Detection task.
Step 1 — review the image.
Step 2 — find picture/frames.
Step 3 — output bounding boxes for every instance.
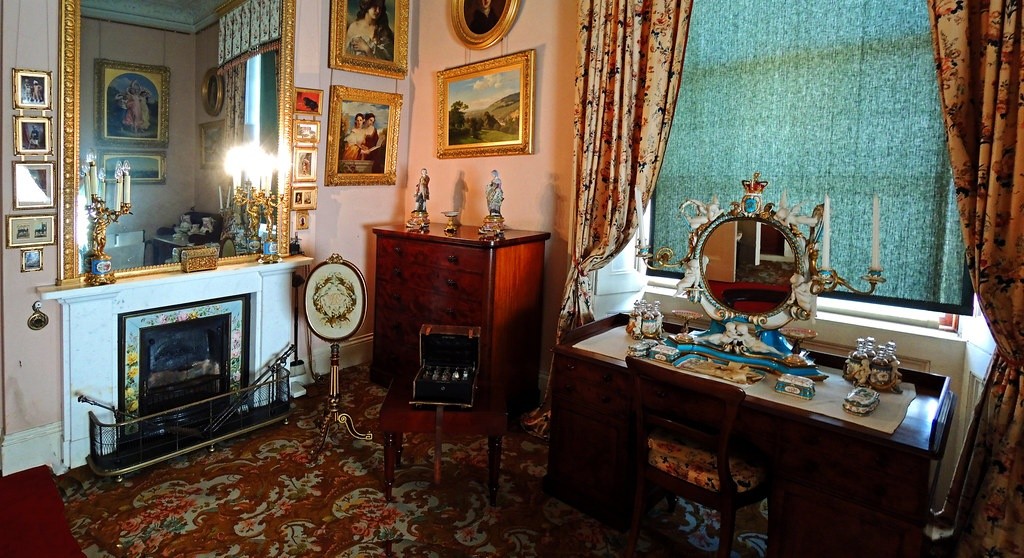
[97,150,167,185]
[201,67,223,116]
[329,0,409,80]
[290,87,324,231]
[434,49,536,160]
[94,58,171,149]
[324,85,404,187]
[449,0,522,50]
[4,68,57,272]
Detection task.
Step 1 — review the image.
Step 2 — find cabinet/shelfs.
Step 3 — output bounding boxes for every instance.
[371,221,552,414]
[153,211,223,265]
[545,310,956,558]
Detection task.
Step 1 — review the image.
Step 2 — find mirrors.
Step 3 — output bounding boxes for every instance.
[673,174,829,330]
[53,0,296,289]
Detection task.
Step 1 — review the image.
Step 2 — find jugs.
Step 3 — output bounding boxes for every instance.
[189,224,200,234]
[180,215,192,232]
[200,217,215,234]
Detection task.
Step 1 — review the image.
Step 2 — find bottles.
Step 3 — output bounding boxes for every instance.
[625,299,664,340]
[842,337,901,389]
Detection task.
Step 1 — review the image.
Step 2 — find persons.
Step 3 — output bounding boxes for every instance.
[302,163,307,174]
[685,204,724,228]
[673,260,700,297]
[414,168,429,212]
[297,193,311,204]
[788,274,816,324]
[633,315,642,334]
[777,202,822,227]
[27,126,39,149]
[23,79,43,102]
[182,192,260,241]
[245,194,276,239]
[890,360,902,382]
[486,171,503,216]
[694,323,784,357]
[856,359,870,384]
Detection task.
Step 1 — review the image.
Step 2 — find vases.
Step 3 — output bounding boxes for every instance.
[199,119,227,170]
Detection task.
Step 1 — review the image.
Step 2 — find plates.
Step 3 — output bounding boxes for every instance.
[441,211,459,217]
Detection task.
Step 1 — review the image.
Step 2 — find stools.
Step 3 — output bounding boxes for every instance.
[380,371,508,507]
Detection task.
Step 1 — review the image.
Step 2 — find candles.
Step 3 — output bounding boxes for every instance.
[219,185,223,210]
[635,186,647,246]
[227,186,230,208]
[871,193,879,268]
[822,194,831,271]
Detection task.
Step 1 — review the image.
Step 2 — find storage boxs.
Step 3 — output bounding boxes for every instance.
[408,323,480,410]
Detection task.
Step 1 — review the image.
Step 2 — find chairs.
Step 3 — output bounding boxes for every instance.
[624,356,768,558]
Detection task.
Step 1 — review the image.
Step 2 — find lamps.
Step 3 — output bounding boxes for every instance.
[79,149,131,286]
[229,155,271,255]
[228,150,284,264]
[82,163,123,274]
